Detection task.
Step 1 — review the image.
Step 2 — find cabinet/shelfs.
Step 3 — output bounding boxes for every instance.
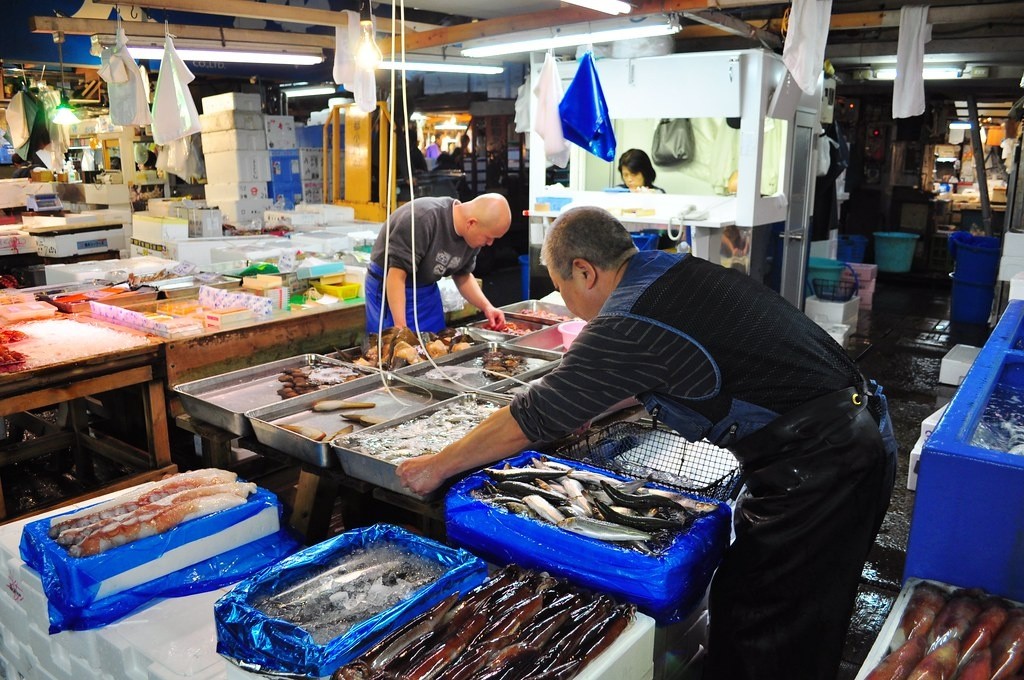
[102,135,170,198]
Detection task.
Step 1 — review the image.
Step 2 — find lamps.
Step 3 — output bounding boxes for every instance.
[281,83,337,97]
[563,0,640,16]
[460,12,683,57]
[52,31,80,125]
[90,33,325,65]
[378,53,504,75]
[870,63,966,78]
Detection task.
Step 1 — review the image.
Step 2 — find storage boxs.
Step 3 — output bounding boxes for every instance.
[0,450,732,680]
[997,229,1024,301]
[855,577,1023,680]
[804,234,878,350]
[908,403,951,491]
[0,92,384,286]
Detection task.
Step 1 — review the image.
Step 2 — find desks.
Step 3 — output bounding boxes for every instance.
[176,410,447,541]
[0,352,178,527]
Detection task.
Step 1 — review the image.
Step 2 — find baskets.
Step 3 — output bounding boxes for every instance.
[309,281,360,300]
[812,279,857,302]
[557,420,745,502]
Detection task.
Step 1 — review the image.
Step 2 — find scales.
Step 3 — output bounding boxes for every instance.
[26,192,64,212]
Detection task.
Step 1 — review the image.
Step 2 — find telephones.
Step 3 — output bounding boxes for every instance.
[666,203,711,222]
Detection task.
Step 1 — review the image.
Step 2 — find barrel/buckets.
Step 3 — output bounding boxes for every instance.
[518,254,530,301]
[804,255,846,297]
[631,232,658,251]
[948,231,1002,281]
[837,234,869,264]
[949,270,998,325]
[874,231,919,273]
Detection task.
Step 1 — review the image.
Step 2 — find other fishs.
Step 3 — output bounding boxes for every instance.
[253,555,436,631]
[955,648,992,680]
[902,583,950,641]
[924,589,983,656]
[863,634,925,680]
[958,605,1010,670]
[330,562,638,680]
[907,637,961,680]
[471,456,718,558]
[989,616,1024,680]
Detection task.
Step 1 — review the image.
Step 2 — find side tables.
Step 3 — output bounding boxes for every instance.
[939,344,982,385]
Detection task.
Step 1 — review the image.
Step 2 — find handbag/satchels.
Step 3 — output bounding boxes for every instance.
[651,118,695,166]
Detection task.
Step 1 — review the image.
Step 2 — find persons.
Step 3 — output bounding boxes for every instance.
[616,149,666,194]
[396,129,477,203]
[365,193,511,347]
[12,153,40,178]
[129,149,165,211]
[395,205,897,680]
